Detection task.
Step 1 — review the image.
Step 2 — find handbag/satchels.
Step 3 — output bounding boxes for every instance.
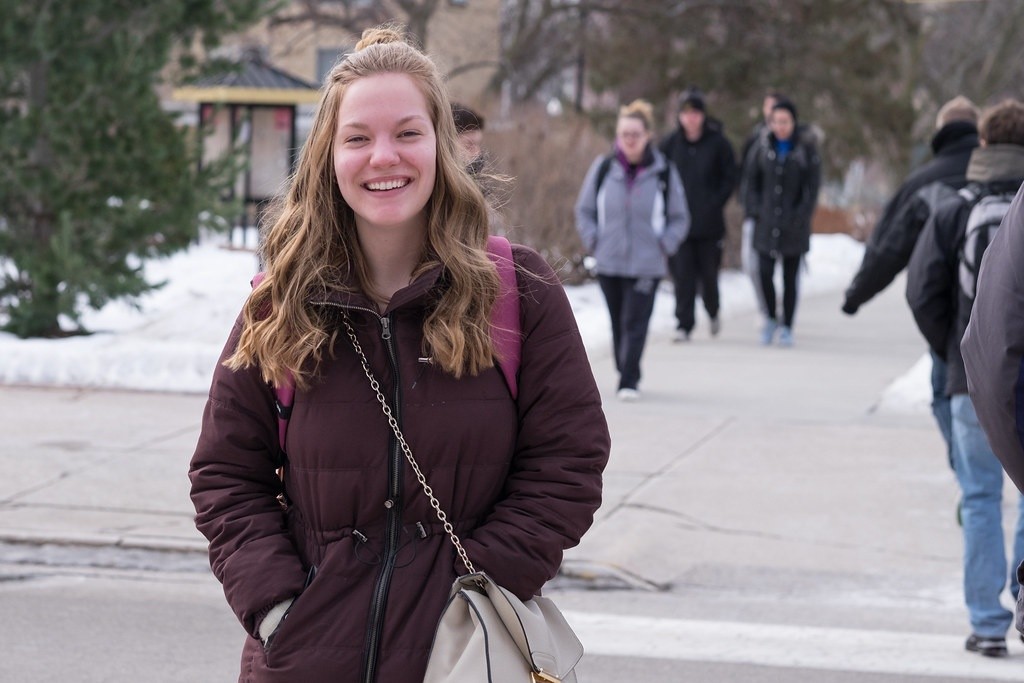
[422,570,584,683]
[736,139,764,203]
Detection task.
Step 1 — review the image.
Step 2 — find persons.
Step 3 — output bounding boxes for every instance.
[188,28,610,682]
[841,91,1024,658]
[447,100,509,243]
[572,87,823,401]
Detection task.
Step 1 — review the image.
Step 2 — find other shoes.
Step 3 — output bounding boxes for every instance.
[619,388,637,400]
[710,315,719,335]
[966,633,1008,657]
[778,323,792,344]
[758,317,777,344]
[957,499,963,526]
[674,328,687,340]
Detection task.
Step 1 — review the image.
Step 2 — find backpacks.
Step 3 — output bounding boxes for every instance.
[958,186,1018,326]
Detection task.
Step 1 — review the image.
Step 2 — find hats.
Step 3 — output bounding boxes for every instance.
[678,89,706,112]
